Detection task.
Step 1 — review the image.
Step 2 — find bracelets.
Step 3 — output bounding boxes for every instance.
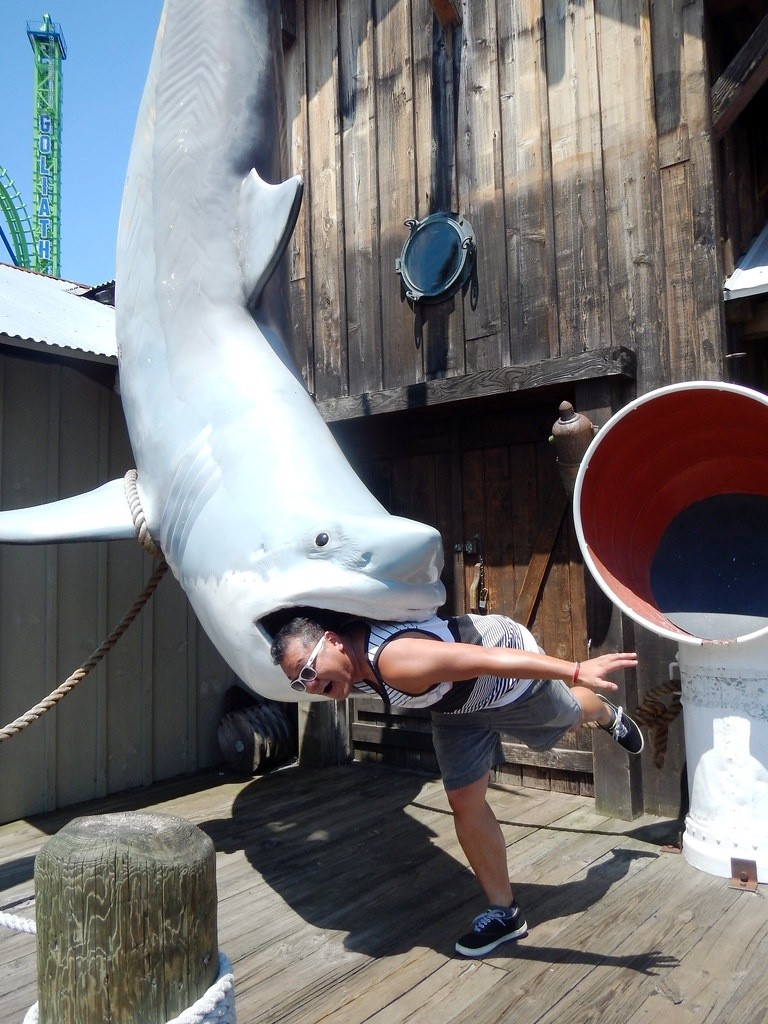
[572,662,580,684]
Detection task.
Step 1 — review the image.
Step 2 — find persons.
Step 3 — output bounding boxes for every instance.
[269,614,645,957]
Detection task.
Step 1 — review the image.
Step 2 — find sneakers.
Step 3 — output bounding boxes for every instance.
[595,692,645,754]
[455,904,529,958]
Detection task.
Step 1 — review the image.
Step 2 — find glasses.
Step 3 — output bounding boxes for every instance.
[290,634,326,695]
